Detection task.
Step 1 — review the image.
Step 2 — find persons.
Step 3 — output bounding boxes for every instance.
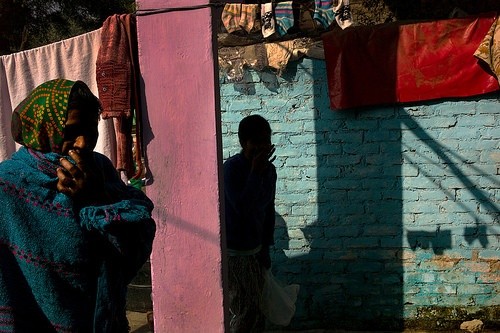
[0,78,157,333]
[223,113,278,333]
